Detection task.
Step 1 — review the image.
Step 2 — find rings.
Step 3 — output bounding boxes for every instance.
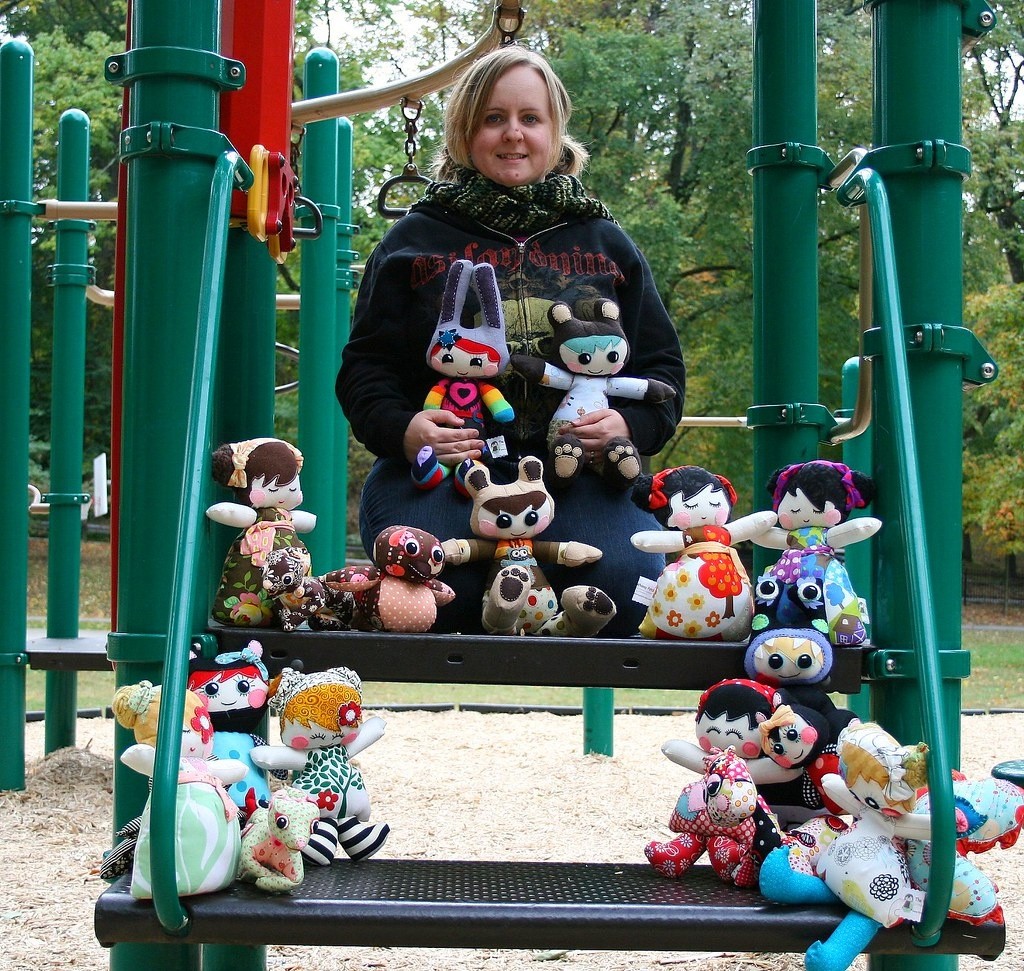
[589,449,597,467]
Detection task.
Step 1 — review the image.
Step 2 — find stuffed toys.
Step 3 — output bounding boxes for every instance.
[205,437,884,642]
[408,259,514,488]
[511,281,678,482]
[645,629,1024,970]
[111,637,392,900]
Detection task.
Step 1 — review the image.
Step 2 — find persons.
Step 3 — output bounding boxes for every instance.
[335,43,686,637]
